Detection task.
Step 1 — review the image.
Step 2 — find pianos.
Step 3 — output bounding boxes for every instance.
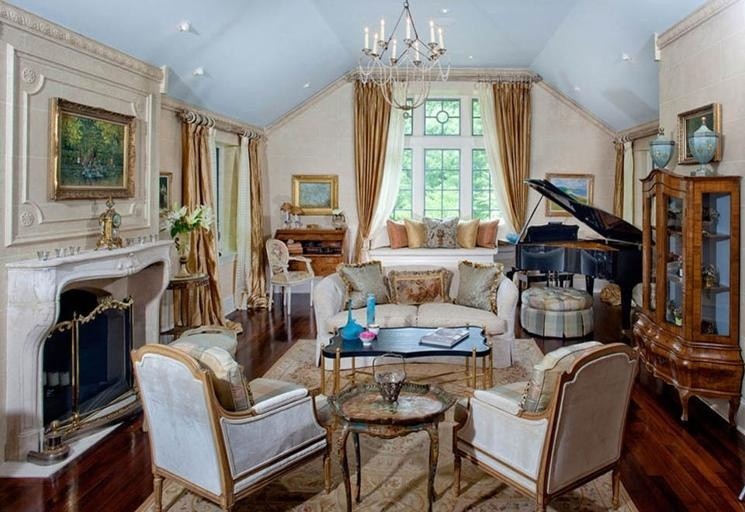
[514,179,655,329]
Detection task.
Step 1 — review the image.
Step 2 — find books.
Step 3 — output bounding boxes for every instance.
[420,327,470,349]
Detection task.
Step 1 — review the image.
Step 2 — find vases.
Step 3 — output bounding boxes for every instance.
[174,258,192,278]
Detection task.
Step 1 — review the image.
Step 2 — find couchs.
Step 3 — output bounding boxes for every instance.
[362,222,513,266]
[314,259,520,370]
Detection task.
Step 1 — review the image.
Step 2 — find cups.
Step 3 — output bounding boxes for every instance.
[38,233,160,262]
[368,324,380,336]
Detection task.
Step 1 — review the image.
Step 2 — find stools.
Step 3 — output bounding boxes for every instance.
[521,287,595,339]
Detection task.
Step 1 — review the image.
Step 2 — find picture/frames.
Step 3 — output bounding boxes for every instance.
[545,173,594,217]
[159,171,173,218]
[292,175,337,215]
[677,103,721,165]
[48,97,137,199]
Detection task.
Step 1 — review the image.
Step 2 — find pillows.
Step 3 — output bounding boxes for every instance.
[336,259,505,315]
[386,216,499,248]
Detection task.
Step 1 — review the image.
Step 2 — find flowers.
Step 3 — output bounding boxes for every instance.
[159,203,215,257]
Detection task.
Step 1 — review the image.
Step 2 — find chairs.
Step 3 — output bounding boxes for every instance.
[453,342,639,511]
[265,239,314,316]
[131,344,334,512]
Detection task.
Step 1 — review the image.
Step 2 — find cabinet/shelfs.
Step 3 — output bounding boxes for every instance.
[633,168,744,428]
[274,229,345,278]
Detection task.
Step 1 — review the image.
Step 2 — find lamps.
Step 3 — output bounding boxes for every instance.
[358,1,451,110]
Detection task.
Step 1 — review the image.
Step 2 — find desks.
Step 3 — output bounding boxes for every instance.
[159,273,210,340]
[326,382,456,512]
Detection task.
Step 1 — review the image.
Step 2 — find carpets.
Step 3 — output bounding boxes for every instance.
[136,339,638,512]
